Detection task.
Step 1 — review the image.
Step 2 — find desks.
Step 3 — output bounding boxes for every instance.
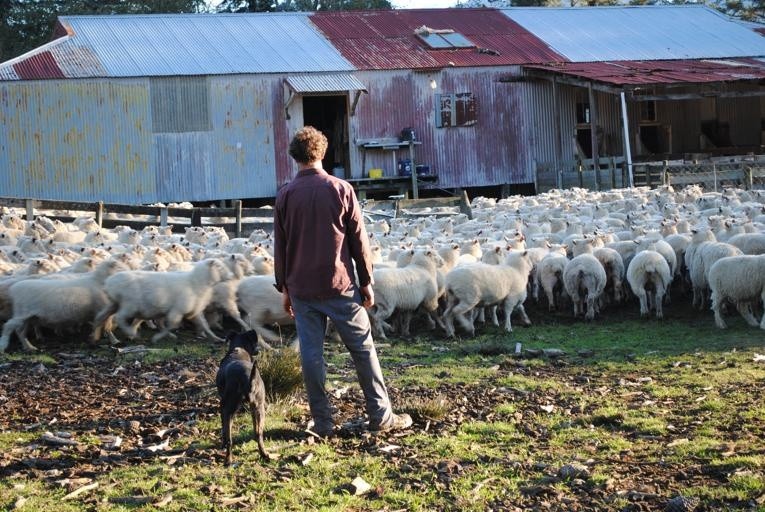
[344,174,439,202]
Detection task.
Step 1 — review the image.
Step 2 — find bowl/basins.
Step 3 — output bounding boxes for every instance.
[369,169,382,178]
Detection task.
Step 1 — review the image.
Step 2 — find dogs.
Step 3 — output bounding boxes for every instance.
[214,328,278,467]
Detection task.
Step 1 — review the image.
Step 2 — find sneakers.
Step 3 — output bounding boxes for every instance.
[370,413,412,434]
[310,426,333,439]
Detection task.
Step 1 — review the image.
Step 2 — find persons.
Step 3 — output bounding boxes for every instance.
[272,126,413,437]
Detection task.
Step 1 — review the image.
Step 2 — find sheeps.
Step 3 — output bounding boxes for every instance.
[1,183,765,353]
